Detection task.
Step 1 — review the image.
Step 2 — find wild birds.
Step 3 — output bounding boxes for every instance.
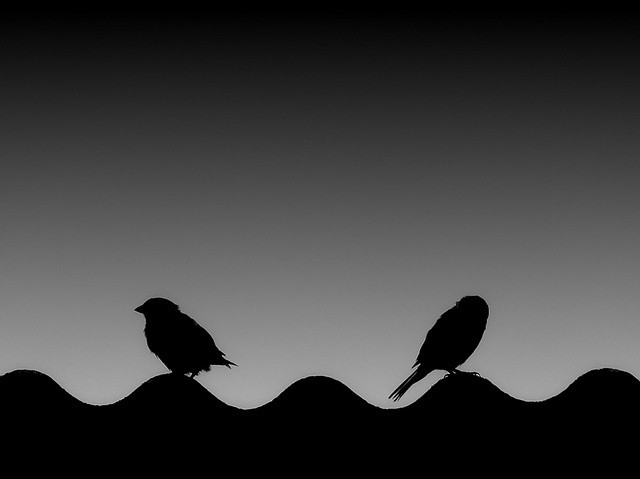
[388,295,489,402]
[133,297,239,379]
